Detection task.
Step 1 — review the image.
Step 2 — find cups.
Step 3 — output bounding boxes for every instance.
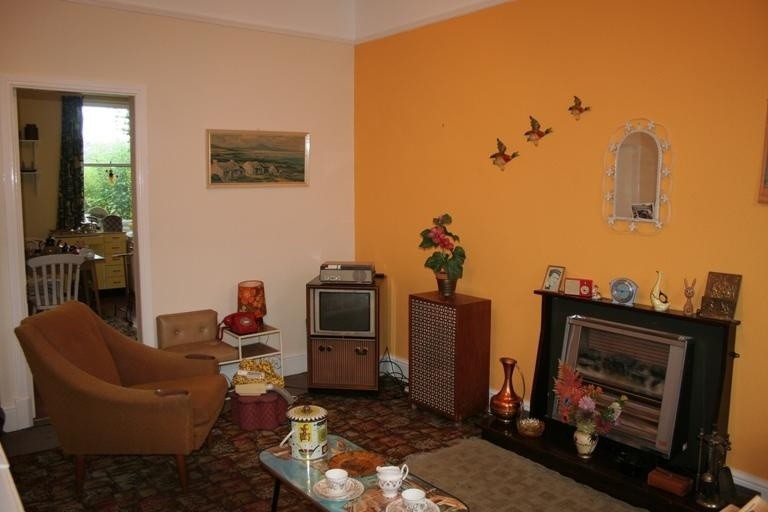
[325,467,347,496]
[402,488,427,512]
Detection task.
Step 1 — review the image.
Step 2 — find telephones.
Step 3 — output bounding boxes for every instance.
[223,311,258,335]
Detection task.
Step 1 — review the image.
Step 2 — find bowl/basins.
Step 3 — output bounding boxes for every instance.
[516,415,545,439]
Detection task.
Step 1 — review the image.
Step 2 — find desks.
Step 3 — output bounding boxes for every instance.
[26,248,103,318]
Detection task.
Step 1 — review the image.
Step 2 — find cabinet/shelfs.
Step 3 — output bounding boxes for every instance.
[305,274,381,393]
[19,138,39,176]
[49,232,129,291]
[406,291,492,425]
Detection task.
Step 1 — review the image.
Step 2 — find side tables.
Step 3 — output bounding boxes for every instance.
[220,323,285,385]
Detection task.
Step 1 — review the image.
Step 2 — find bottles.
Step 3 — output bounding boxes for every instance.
[56,241,80,256]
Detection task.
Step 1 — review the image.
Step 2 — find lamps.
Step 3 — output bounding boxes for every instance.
[238,279,267,326]
[693,421,732,508]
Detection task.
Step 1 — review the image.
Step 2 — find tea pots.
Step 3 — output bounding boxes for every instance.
[376,464,409,499]
[38,236,56,255]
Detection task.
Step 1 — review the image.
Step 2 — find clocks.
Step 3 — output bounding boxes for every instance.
[608,278,639,308]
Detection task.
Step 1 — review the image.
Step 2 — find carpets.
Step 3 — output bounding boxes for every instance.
[398,436,642,511]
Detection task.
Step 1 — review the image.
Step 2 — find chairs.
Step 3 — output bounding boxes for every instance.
[25,237,47,256]
[27,254,86,315]
[156,308,240,390]
[14,300,226,503]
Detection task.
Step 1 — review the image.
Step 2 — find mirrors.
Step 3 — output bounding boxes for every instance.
[601,118,671,233]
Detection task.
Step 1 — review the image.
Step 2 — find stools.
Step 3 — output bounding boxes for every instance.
[232,390,290,434]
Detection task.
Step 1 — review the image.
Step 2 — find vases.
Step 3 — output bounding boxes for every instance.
[487,356,524,422]
[574,420,600,459]
[432,269,459,299]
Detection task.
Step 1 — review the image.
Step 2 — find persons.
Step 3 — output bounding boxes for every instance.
[543,269,560,291]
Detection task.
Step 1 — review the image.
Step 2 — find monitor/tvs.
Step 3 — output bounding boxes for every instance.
[310,287,376,339]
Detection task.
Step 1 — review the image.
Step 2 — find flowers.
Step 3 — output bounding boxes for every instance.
[419,212,467,279]
[553,360,629,437]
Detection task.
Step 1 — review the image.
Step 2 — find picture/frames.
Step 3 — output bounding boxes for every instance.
[207,128,311,188]
[540,264,566,295]
[757,99,768,204]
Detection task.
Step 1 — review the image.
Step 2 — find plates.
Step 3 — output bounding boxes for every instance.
[311,477,364,501]
[383,497,442,512]
